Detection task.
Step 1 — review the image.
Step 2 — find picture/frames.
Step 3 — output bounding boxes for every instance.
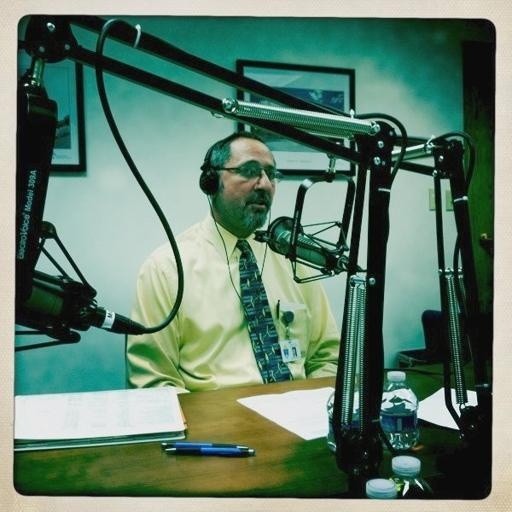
[17,41,90,176]
[234,57,357,179]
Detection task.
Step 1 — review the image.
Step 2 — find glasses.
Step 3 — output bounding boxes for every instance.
[214,167,276,179]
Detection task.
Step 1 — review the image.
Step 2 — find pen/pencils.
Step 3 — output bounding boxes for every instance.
[161,442,255,456]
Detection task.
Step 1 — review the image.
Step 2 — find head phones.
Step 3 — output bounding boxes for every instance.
[199,146,219,195]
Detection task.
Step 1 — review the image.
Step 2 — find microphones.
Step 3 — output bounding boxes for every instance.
[266,216,338,270]
[14,268,96,342]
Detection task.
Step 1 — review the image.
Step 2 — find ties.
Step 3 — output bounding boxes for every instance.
[237,240,290,384]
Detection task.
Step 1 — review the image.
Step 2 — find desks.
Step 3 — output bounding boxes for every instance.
[13,365,492,500]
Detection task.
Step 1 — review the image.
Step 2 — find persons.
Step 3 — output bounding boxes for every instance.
[125,131,343,395]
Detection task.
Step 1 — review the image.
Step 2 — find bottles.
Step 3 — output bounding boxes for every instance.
[327,372,363,453]
[367,454,434,500]
[379,370,422,451]
